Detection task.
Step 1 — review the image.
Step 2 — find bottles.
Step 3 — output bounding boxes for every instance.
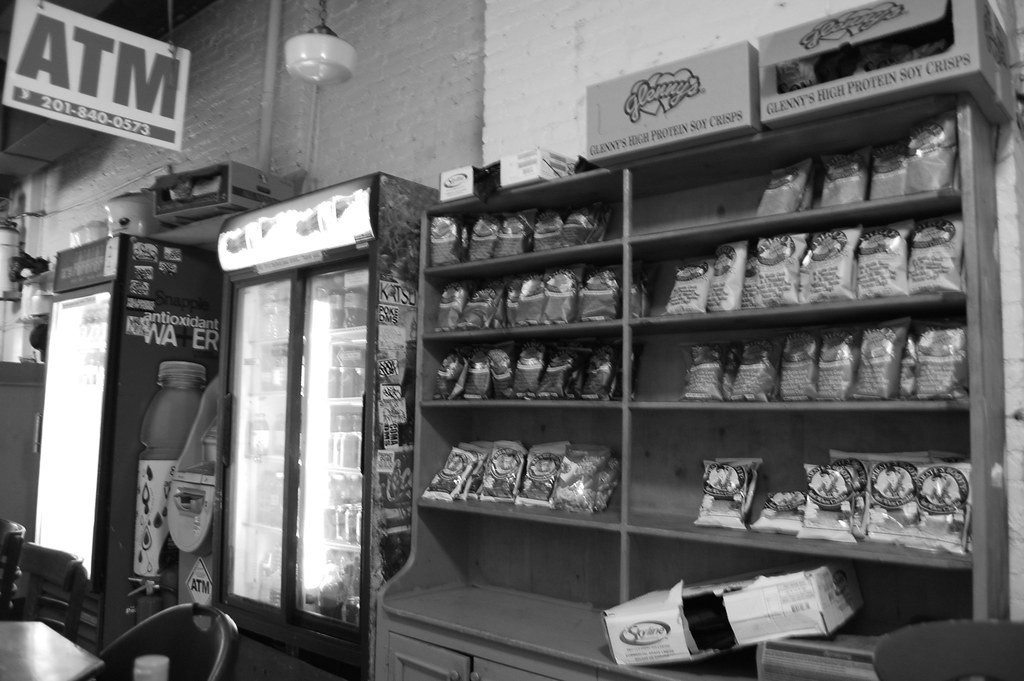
[132,361,206,597]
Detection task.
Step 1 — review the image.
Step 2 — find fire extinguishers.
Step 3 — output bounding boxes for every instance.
[127,576,177,624]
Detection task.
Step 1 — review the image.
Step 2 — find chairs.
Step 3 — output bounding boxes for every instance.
[88,601,240,681]
[9,542,87,643]
[0,516,27,620]
[872,617,1024,681]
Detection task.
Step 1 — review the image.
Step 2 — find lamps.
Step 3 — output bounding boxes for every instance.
[284,0,361,86]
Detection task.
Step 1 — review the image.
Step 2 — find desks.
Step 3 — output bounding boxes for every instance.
[0,620,106,681]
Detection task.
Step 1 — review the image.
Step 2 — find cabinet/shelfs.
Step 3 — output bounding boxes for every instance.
[368,90,1011,681]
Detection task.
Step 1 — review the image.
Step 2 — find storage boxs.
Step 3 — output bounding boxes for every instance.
[601,558,865,666]
[148,159,295,227]
[439,164,498,203]
[753,618,889,681]
[586,39,761,168]
[758,0,1016,127]
[500,146,579,189]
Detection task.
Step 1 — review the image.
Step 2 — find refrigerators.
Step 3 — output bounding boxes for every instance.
[34,233,220,653]
[217,172,438,681]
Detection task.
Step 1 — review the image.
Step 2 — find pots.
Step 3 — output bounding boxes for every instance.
[104,192,155,237]
